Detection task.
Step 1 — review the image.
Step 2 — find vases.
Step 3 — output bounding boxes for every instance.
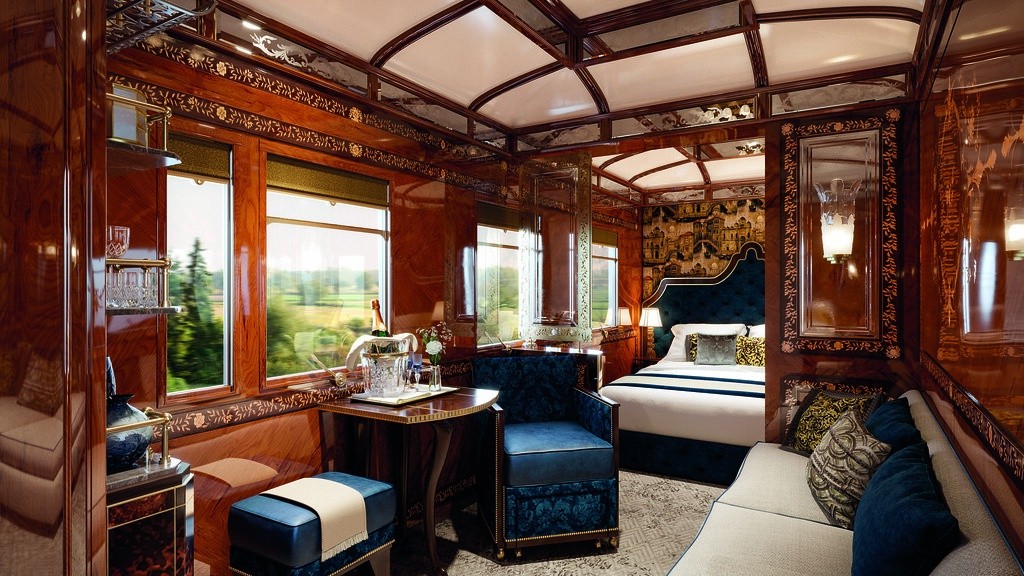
[428,364,440,391]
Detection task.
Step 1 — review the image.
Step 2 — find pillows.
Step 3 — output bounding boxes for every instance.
[849,440,970,576]
[863,396,925,457]
[778,384,876,460]
[665,323,765,368]
[802,406,891,529]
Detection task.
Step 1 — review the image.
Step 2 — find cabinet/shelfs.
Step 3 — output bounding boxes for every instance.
[104,134,198,576]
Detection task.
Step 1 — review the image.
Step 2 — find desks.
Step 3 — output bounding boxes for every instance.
[314,380,500,567]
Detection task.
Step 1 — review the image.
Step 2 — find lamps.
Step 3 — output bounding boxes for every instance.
[810,175,863,262]
[617,306,632,325]
[638,307,663,360]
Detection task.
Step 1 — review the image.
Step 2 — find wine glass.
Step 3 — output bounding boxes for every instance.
[405,351,414,388]
[412,354,422,392]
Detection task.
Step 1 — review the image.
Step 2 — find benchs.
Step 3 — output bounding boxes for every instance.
[226,470,396,576]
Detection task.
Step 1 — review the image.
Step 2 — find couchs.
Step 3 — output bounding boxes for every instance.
[664,388,1022,576]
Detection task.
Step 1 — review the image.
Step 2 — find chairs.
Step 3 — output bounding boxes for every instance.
[469,352,621,563]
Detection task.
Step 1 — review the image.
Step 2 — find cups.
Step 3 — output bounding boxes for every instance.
[106,225,130,257]
[106,271,158,308]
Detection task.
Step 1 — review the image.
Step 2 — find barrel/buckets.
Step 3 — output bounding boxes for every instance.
[360,351,407,396]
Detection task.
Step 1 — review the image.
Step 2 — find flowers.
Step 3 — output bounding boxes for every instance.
[415,320,451,386]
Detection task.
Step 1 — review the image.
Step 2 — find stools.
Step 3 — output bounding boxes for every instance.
[188,456,278,513]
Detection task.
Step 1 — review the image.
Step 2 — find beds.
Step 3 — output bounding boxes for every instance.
[595,242,765,459]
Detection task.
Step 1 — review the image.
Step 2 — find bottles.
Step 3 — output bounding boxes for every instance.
[370,299,394,353]
